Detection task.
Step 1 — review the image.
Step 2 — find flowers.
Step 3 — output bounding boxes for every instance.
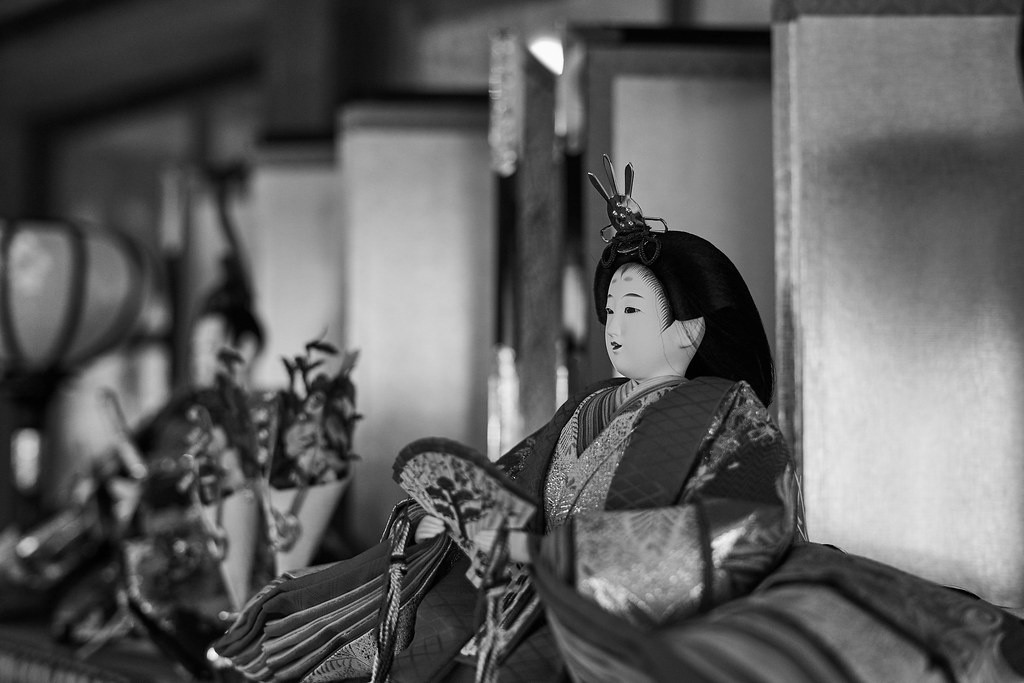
[183,331,363,567]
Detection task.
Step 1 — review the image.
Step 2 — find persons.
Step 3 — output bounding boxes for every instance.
[208,231,1024,683]
